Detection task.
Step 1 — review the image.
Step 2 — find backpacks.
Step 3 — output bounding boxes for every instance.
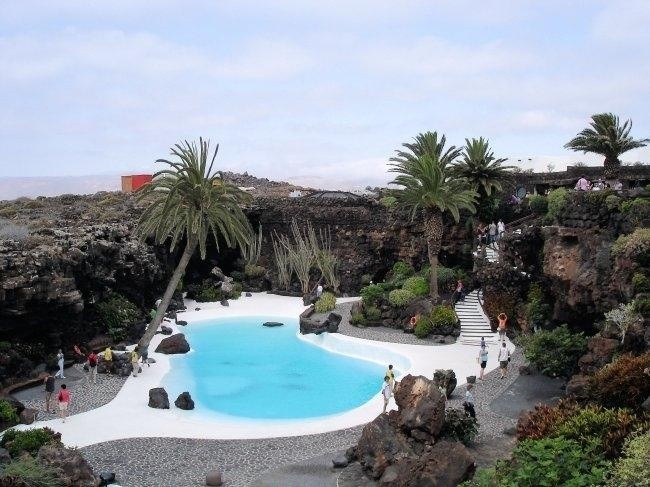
[89,355,96,367]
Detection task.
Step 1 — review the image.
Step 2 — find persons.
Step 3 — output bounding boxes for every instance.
[456,279,464,301]
[142,342,150,367]
[143,297,163,331]
[317,283,323,296]
[72,343,98,384]
[53,349,66,379]
[57,384,70,423]
[46,371,56,413]
[477,311,511,381]
[104,342,113,377]
[505,193,521,205]
[574,173,623,197]
[382,365,397,415]
[465,384,478,423]
[131,346,140,378]
[477,218,506,244]
[431,375,450,420]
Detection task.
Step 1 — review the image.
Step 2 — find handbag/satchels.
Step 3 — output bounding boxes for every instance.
[59,392,62,401]
